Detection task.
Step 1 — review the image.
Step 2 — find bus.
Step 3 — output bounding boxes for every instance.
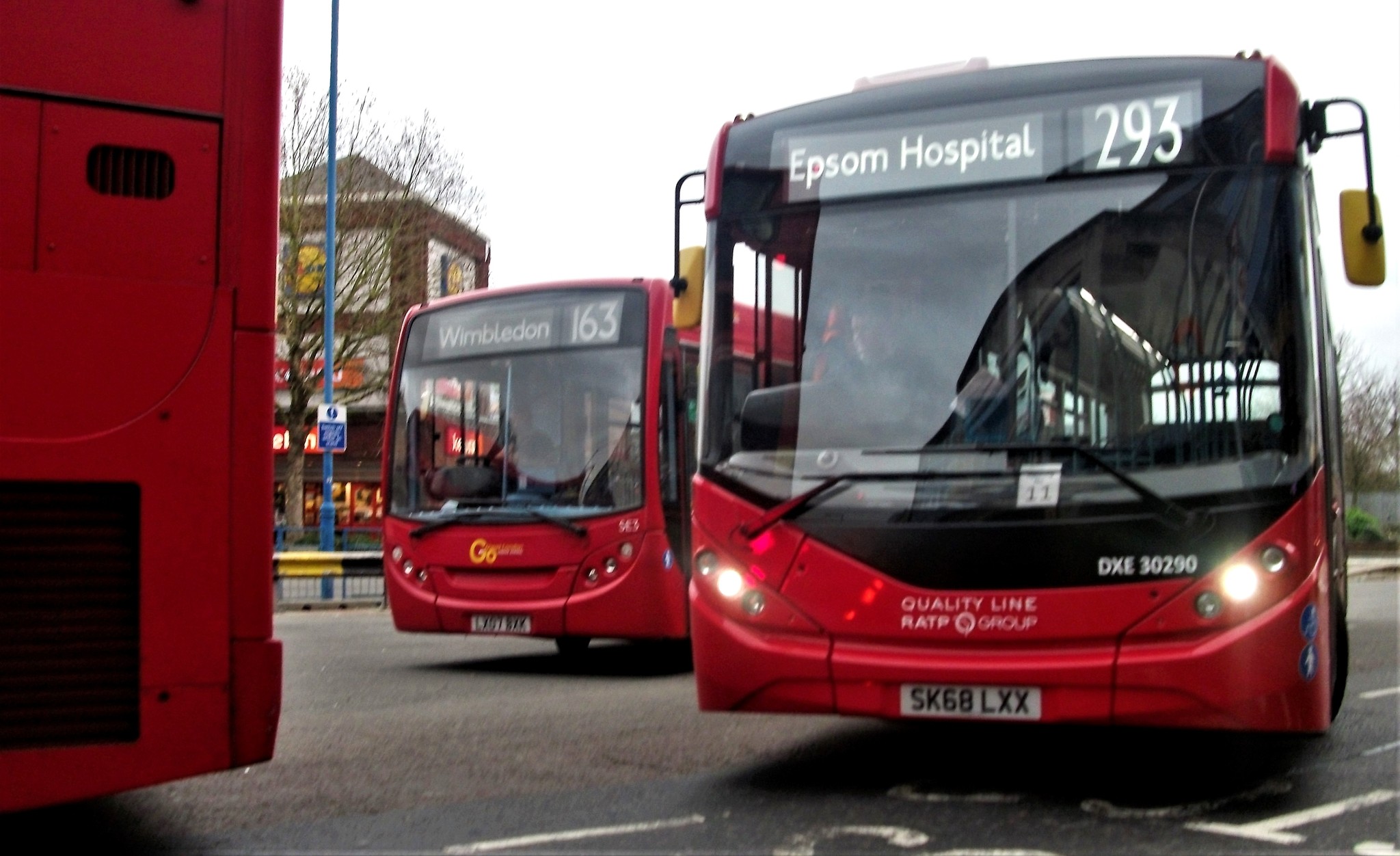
[380,277,809,656]
[0,0,284,813]
[668,48,1388,736]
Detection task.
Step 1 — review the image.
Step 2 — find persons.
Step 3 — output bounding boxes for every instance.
[828,296,954,447]
[482,355,564,497]
